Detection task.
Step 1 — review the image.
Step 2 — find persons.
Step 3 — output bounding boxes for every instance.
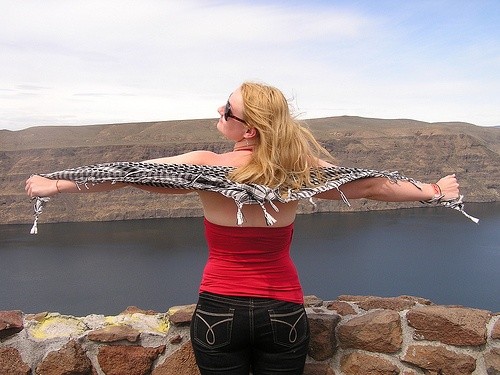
[23,82,461,375]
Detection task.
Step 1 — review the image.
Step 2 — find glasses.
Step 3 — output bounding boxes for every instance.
[224,99,247,126]
